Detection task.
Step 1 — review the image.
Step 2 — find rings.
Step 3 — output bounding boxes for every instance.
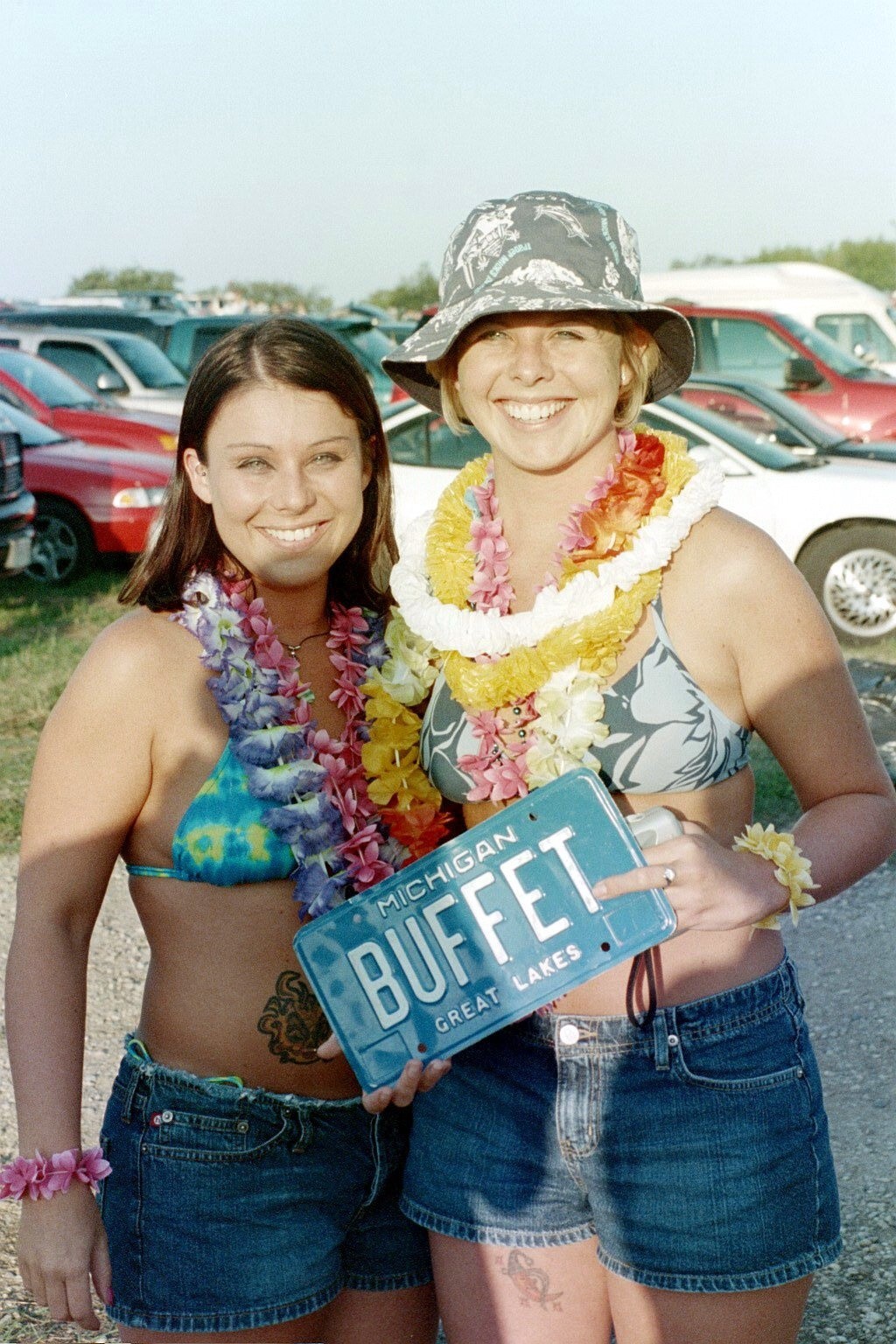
[663,865,677,889]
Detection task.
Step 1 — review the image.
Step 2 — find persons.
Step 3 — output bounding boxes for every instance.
[384,191,896,1344]
[2,318,442,1344]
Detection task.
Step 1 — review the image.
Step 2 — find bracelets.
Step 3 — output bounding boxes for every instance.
[732,821,821,931]
[0,1147,113,1202]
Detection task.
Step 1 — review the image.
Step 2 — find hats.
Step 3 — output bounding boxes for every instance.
[380,191,695,425]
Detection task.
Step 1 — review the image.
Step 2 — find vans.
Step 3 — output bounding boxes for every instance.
[654,256,895,381]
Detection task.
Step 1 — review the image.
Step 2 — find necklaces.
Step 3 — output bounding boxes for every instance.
[280,629,331,659]
[386,418,724,806]
[173,549,454,916]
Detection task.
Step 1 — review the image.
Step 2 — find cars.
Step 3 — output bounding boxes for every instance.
[1,287,428,412]
[1,344,187,590]
[388,369,895,464]
[419,294,895,450]
[374,392,895,651]
